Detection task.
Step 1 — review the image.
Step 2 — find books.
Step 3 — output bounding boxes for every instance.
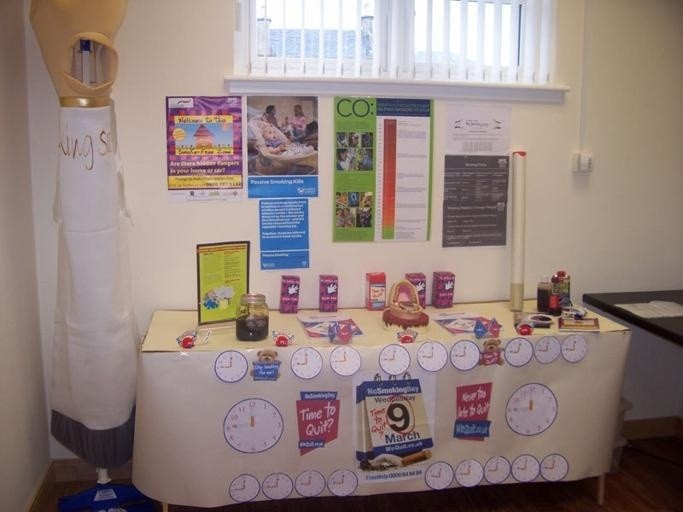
[557,315,600,332]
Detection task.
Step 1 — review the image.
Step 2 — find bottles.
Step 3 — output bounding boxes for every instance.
[236,293,270,342]
[536,269,571,316]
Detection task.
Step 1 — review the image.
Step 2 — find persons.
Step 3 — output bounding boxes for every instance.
[260,126,314,158]
[283,103,308,139]
[260,103,278,128]
[336,208,344,227]
[338,207,355,229]
[336,148,349,171]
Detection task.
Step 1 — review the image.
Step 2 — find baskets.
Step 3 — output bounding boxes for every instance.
[390,280,422,320]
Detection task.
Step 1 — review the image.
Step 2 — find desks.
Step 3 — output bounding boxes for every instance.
[131,299,632,512]
[583,290,683,348]
